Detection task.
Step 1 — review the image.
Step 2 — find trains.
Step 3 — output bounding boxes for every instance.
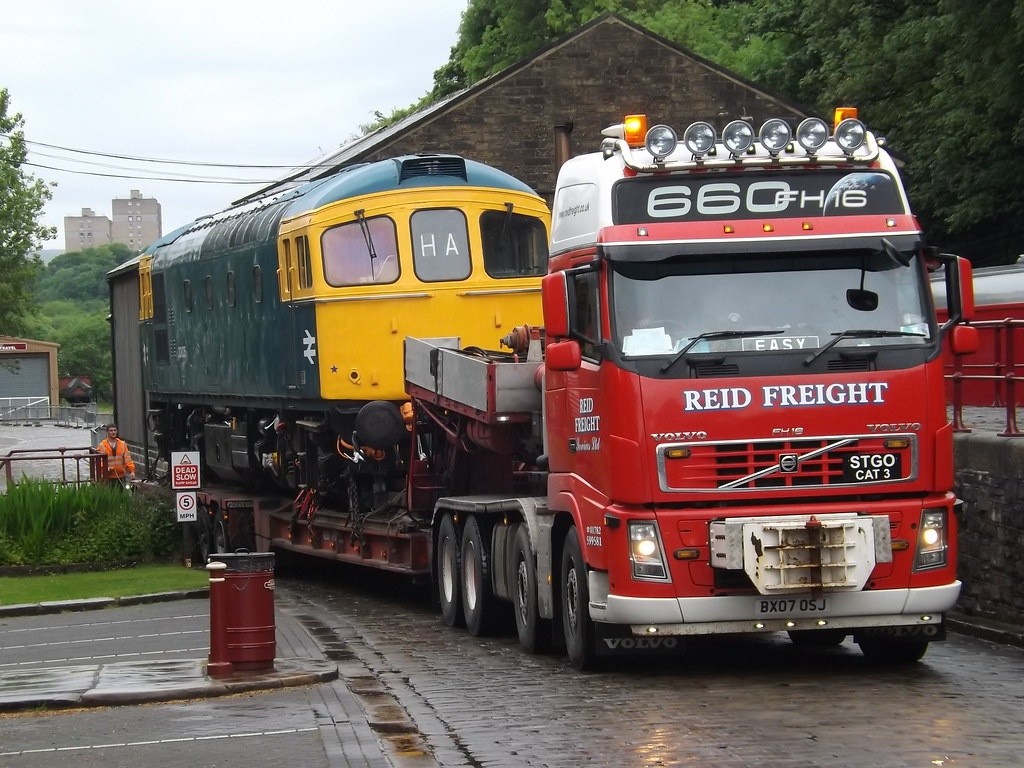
[135,148,552,516]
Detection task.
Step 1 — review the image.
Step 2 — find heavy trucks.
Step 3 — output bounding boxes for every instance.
[139,105,979,678]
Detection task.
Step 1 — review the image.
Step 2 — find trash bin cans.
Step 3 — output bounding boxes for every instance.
[208,547,277,678]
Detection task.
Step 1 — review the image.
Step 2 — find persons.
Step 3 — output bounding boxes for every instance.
[96,423,136,493]
[636,318,649,328]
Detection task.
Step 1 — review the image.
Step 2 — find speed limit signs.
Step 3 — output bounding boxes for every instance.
[177,492,198,522]
